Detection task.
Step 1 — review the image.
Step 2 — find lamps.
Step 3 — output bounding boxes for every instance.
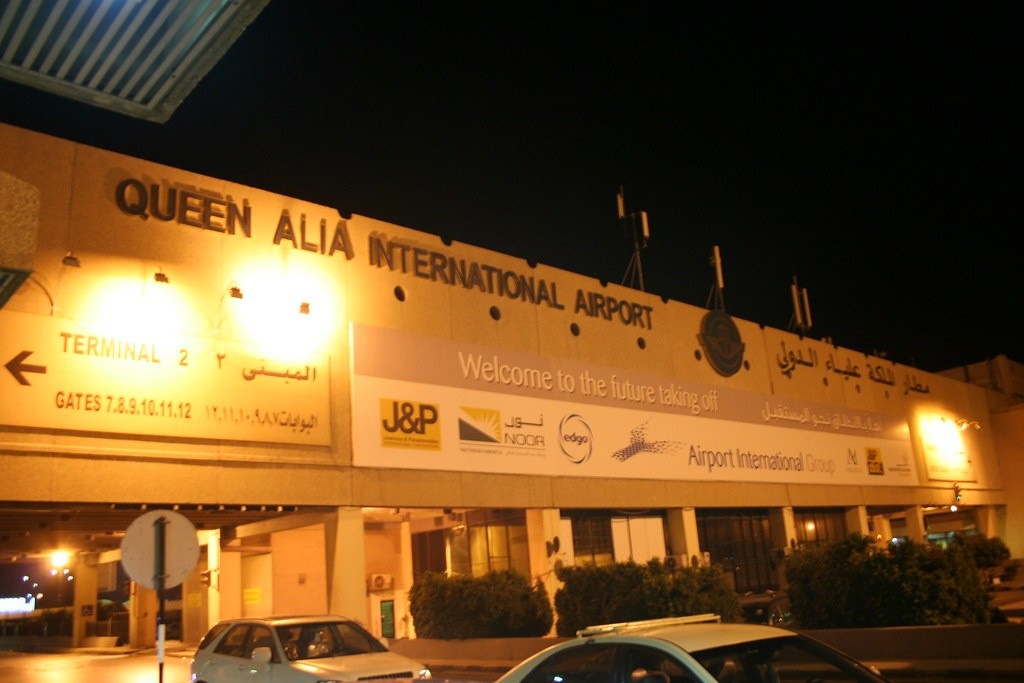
[155,272,169,282]
[62,256,81,267]
[230,288,243,299]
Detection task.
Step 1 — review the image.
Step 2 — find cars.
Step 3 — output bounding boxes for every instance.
[190,614,433,683]
[495,614,897,682]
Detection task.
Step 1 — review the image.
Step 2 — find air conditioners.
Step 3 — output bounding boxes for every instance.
[770,546,789,563]
[666,553,687,568]
[372,574,392,590]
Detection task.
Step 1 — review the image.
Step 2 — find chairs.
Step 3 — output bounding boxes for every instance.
[305,631,330,658]
[274,632,298,663]
